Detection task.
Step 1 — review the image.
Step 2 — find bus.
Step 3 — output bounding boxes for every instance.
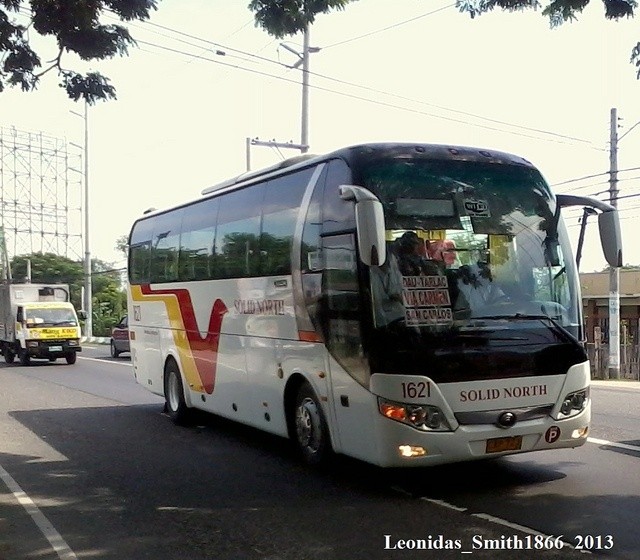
[127,142,622,471]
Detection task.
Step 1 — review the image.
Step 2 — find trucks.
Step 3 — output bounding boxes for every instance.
[1,284,81,367]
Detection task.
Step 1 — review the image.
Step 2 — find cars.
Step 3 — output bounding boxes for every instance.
[109,312,130,358]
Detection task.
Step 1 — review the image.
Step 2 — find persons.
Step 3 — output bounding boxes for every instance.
[395,231,430,276]
[369,253,420,334]
[453,263,510,311]
